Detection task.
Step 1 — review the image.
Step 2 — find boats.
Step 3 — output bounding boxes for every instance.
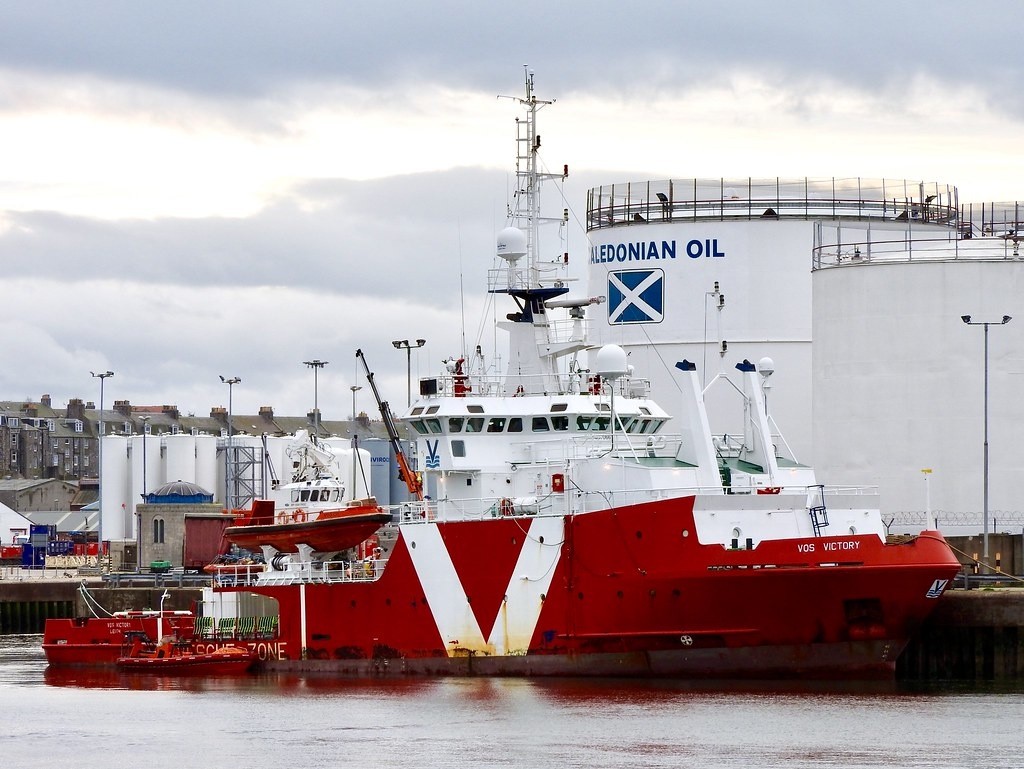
[115,588,261,675]
[222,424,393,553]
[202,63,962,676]
[42,608,196,667]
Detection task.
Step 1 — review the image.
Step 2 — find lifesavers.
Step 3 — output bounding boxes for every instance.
[278,510,288,524]
[501,498,514,516]
[292,509,305,523]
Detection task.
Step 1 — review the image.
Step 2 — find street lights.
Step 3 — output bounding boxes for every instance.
[392,339,426,501]
[963,314,1011,569]
[302,359,329,478]
[218,375,240,515]
[350,386,362,500]
[139,416,152,504]
[90,371,114,554]
